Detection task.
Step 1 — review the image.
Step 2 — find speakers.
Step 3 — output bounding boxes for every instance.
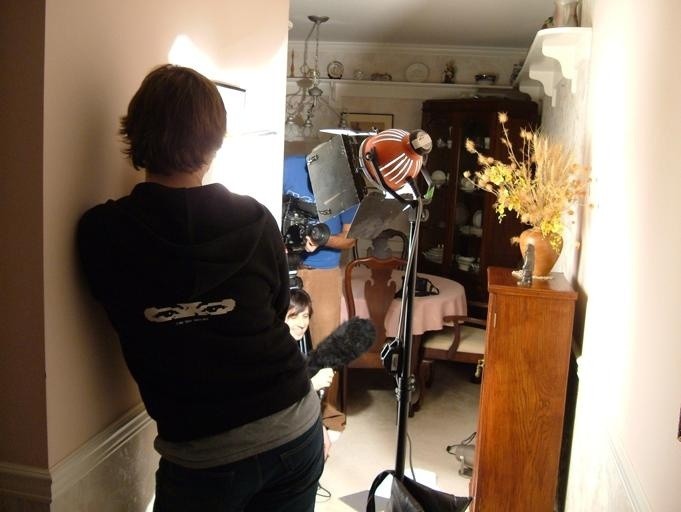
[304,135,363,223]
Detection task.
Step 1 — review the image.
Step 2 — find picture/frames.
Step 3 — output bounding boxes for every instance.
[340,112,397,133]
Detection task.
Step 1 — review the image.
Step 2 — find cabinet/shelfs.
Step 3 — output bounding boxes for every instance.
[466,266,580,511]
[417,98,540,329]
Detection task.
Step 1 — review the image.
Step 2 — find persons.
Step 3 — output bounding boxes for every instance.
[73,62,325,511]
[283,154,361,443]
[284,288,336,464]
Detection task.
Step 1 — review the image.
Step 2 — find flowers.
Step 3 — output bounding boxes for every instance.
[464,106,597,260]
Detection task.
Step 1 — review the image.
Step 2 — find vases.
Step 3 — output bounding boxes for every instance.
[517,228,565,278]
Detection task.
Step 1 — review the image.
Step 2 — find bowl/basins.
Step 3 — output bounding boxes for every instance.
[474,74,497,85]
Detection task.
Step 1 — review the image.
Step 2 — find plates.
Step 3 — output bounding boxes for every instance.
[326,61,344,79]
[405,63,427,83]
[455,203,482,236]
[425,247,444,261]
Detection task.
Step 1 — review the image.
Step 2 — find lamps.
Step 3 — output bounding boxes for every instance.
[360,126,440,511]
[284,14,352,138]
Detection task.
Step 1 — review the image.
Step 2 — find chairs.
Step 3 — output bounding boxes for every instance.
[341,228,486,427]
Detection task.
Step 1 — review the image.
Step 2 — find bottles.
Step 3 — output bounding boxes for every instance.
[521,244,535,287]
